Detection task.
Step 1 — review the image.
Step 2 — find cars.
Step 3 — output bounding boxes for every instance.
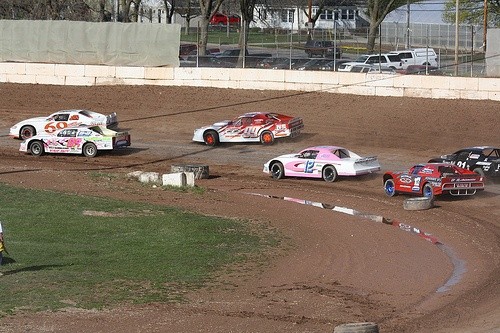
[179,43,404,75]
[262,146,382,183]
[427,146,500,183]
[382,162,486,201]
[192,111,305,147]
[19,123,131,157]
[209,11,241,27]
[8,108,119,140]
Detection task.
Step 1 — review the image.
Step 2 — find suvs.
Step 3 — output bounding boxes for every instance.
[304,39,341,59]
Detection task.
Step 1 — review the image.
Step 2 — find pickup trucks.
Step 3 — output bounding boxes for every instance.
[387,47,438,70]
